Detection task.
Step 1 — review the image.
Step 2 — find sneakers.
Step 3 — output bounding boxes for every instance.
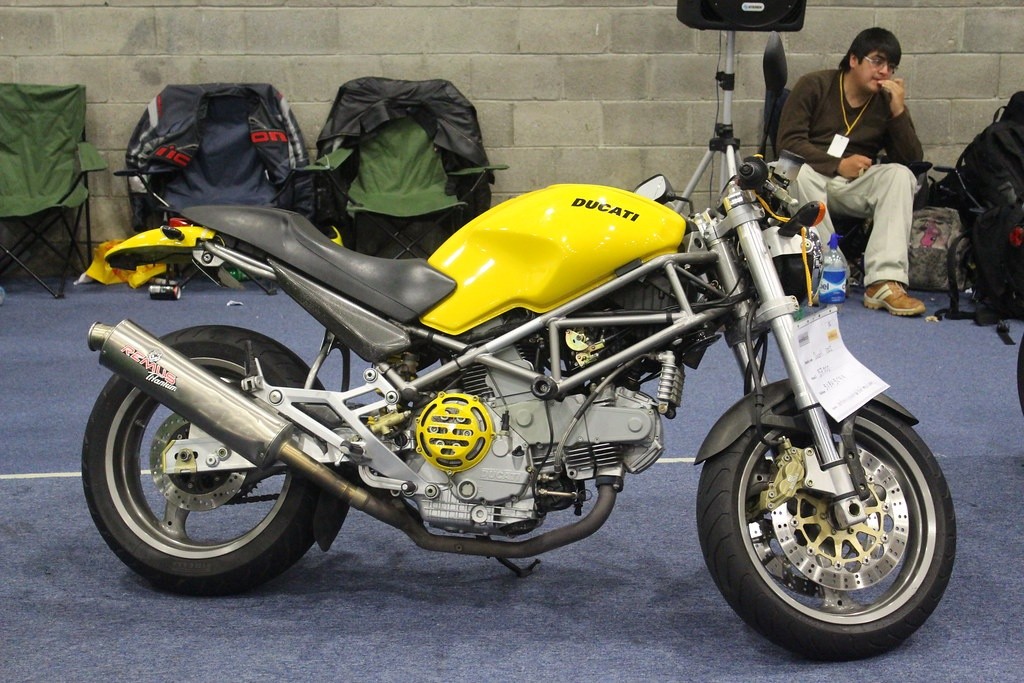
[864,282,926,316]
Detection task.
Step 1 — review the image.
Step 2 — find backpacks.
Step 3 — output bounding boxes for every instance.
[908,206,970,291]
[935,90,1024,344]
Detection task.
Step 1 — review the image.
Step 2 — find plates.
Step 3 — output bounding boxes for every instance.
[230,269,249,282]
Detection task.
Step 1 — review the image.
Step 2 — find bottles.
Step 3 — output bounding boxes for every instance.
[818,234,847,304]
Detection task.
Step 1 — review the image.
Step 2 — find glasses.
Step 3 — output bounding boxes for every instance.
[863,56,899,73]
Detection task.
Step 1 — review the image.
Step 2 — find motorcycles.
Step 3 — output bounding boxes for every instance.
[77,148,959,664]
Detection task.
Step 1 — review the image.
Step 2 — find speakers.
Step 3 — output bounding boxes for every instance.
[676,0,807,31]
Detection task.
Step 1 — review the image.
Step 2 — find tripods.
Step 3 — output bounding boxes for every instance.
[675,31,744,216]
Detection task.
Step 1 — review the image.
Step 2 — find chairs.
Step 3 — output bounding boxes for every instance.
[0,82,109,299]
[112,81,315,298]
[304,76,510,260]
[763,87,933,277]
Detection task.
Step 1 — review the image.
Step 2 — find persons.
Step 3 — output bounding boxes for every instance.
[768,27,926,317]
